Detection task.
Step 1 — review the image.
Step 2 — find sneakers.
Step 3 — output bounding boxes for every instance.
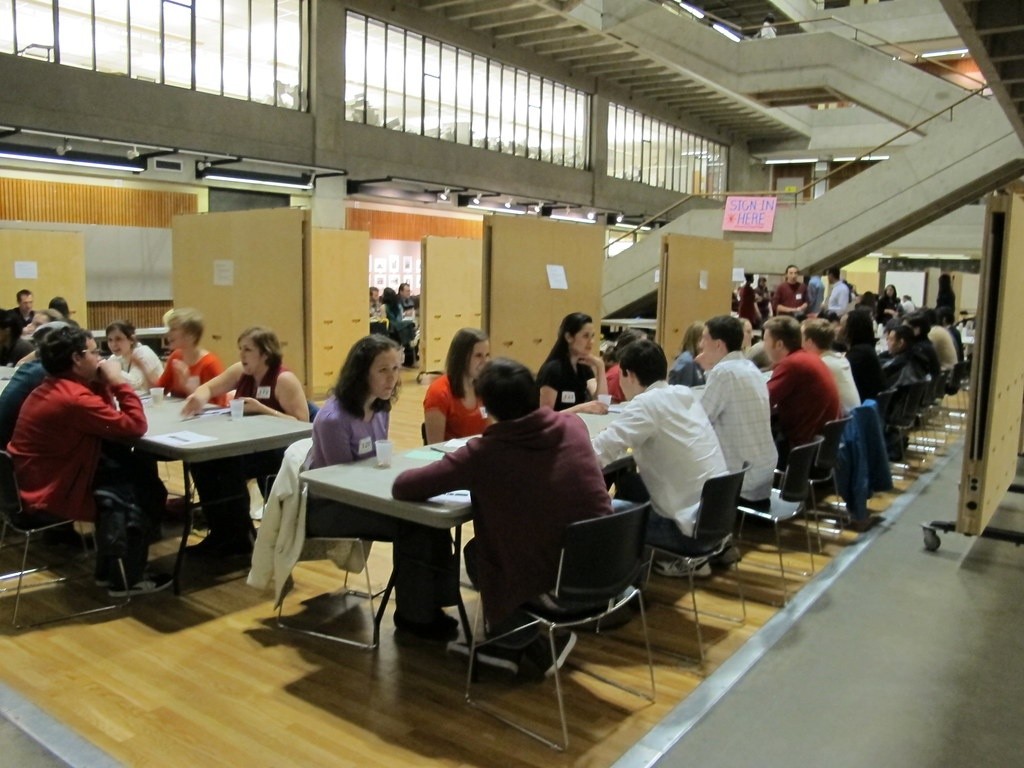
[94,571,110,586]
[713,546,741,565]
[448,640,520,677]
[107,570,173,596]
[537,630,577,678]
[655,555,713,578]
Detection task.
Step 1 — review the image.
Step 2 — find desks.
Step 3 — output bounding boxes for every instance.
[301,388,704,684]
[129,388,313,594]
[89,325,168,346]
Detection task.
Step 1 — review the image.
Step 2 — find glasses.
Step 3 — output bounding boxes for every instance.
[79,348,101,358]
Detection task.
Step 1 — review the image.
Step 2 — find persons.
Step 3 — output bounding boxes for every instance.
[537,313,610,415]
[389,357,616,648]
[0,289,308,596]
[371,283,416,329]
[606,265,964,577]
[593,340,725,538]
[761,13,776,39]
[310,332,460,633]
[424,328,493,443]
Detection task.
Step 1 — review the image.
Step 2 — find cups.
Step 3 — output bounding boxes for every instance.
[375,439,392,469]
[229,399,245,421]
[597,394,612,406]
[148,387,164,407]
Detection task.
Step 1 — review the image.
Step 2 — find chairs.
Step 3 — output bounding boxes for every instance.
[464,353,973,750]
[0,451,130,629]
[265,474,386,649]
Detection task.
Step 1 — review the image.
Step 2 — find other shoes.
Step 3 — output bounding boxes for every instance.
[186,528,253,559]
[393,609,459,637]
[39,528,71,546]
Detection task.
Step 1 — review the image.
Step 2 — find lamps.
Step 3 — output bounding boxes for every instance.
[441,187,626,223]
[56,136,212,171]
